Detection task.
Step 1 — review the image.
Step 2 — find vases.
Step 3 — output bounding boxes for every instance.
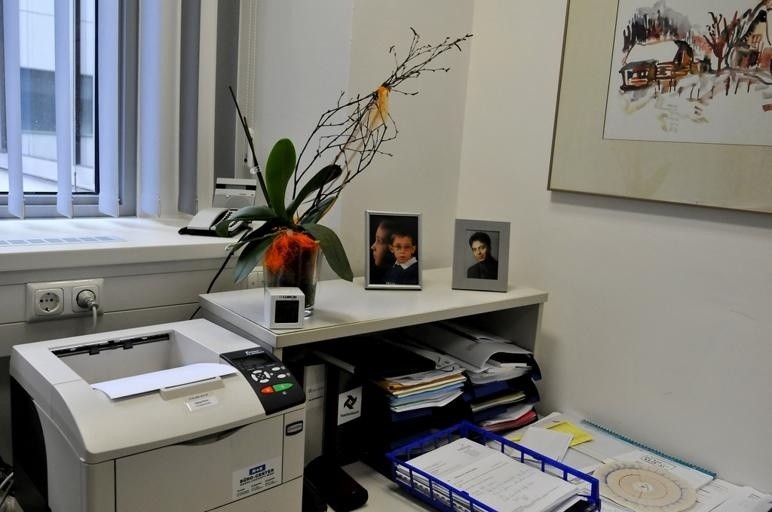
[261,241,323,320]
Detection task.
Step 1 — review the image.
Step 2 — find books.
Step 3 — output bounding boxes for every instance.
[378,327,538,434]
[396,436,578,511]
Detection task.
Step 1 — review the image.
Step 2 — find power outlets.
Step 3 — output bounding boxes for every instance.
[25,278,103,324]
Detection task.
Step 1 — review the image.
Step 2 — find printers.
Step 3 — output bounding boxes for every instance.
[9,319,307,512]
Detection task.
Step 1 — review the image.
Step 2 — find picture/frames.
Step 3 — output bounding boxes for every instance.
[545,0,772,216]
[364,205,511,293]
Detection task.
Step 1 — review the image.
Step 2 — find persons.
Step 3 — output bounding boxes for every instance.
[465,232,497,279]
[377,228,416,284]
[370,218,397,267]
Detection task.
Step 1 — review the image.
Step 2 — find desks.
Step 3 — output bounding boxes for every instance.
[199,265,550,366]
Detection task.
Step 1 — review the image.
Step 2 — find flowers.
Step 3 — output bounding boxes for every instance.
[214,28,474,302]
